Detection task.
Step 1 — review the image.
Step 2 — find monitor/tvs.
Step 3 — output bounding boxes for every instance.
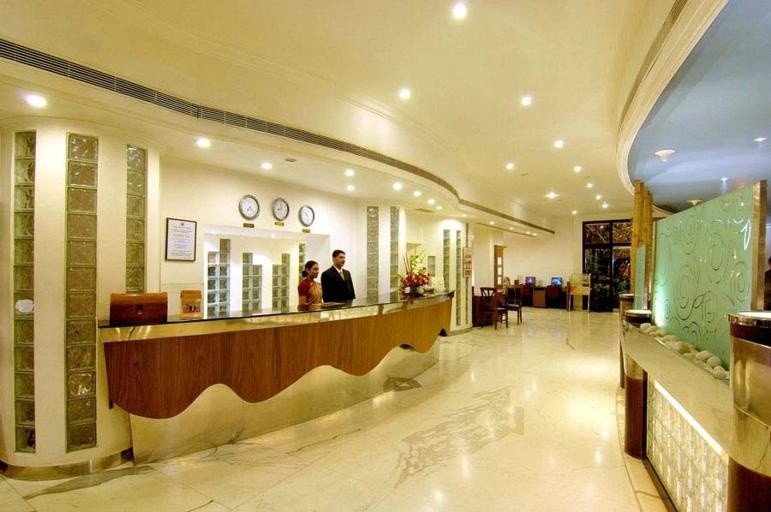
[551,277,562,287]
[525,276,536,285]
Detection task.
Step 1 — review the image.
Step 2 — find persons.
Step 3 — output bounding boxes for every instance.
[297,260,323,311]
[320,250,356,308]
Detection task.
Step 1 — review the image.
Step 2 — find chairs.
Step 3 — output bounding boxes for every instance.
[479,285,561,330]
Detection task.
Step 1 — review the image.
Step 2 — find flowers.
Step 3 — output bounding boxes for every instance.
[396,247,431,299]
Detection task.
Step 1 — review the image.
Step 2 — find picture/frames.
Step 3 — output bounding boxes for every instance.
[165,217,197,261]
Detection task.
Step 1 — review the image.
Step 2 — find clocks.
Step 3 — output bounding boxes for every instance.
[239,194,260,220]
[298,205,314,226]
[272,197,289,221]
